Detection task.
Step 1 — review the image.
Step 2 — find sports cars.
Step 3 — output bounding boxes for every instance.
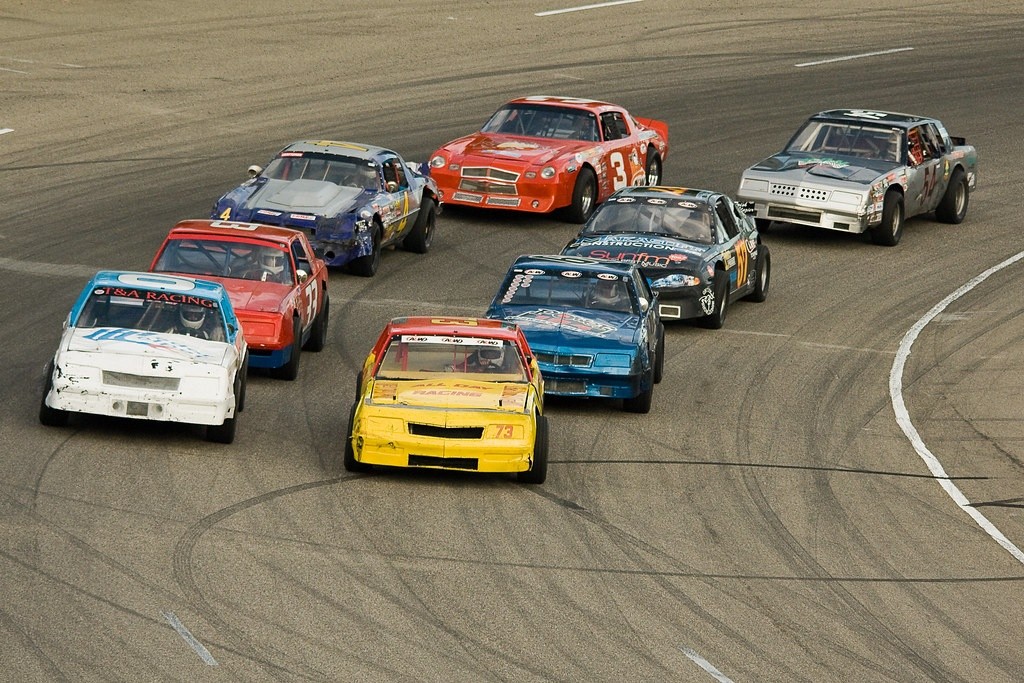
[558,183,771,330]
[733,106,978,247]
[145,216,330,382]
[480,253,668,413]
[210,138,442,278]
[343,314,549,486]
[36,268,252,444]
[426,94,669,225]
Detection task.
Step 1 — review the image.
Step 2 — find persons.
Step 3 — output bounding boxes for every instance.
[165,304,210,340]
[568,117,597,140]
[349,166,397,192]
[592,280,625,310]
[240,247,307,284]
[478,346,504,373]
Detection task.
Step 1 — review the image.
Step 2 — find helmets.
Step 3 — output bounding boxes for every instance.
[592,279,621,306]
[359,164,377,179]
[179,303,206,330]
[478,345,505,369]
[256,246,285,275]
